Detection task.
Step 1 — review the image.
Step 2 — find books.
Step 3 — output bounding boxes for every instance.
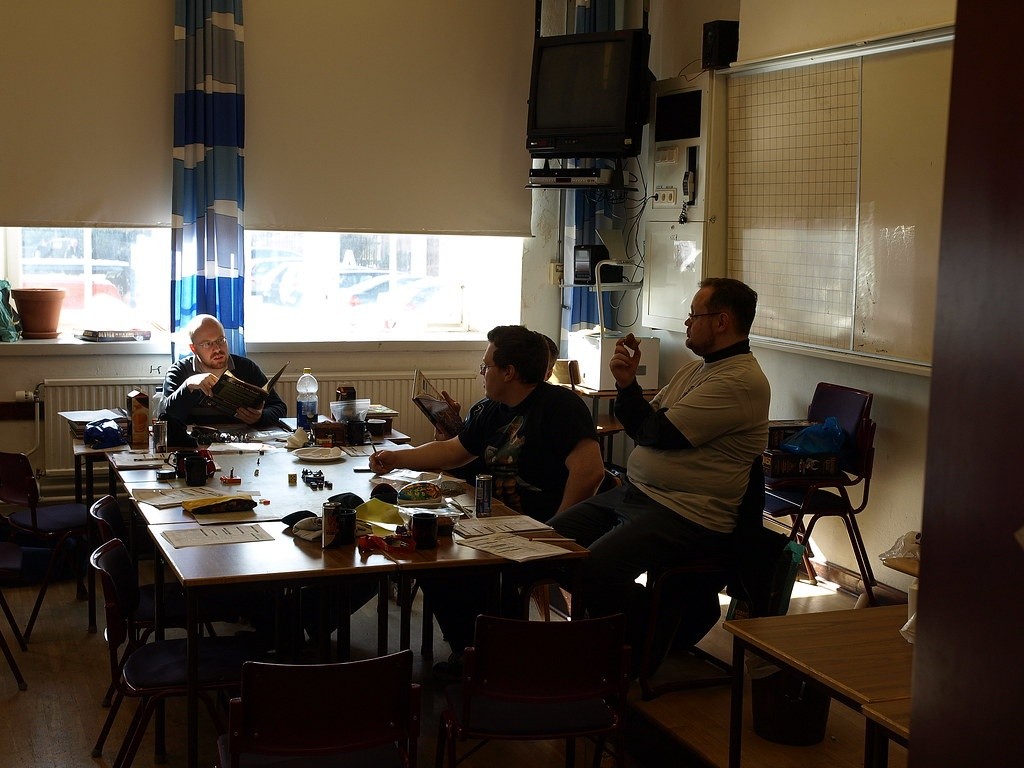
[762,419,848,491]
[411,368,465,438]
[57,408,134,439]
[197,360,290,419]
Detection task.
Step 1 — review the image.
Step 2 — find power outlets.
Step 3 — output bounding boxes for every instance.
[654,188,677,205]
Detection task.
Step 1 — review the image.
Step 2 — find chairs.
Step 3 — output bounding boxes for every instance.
[433,612,630,768]
[500,469,623,619]
[547,359,624,479]
[217,647,422,768]
[635,455,765,702]
[0,451,88,642]
[0,540,29,691]
[89,538,254,768]
[89,494,219,759]
[762,380,876,605]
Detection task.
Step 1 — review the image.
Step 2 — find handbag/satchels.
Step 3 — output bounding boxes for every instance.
[784,415,842,454]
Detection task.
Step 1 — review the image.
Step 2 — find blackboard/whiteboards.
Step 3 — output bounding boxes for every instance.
[714,20,955,378]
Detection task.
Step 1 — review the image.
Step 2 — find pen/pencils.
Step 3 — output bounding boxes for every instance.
[134,458,167,461]
[363,427,387,470]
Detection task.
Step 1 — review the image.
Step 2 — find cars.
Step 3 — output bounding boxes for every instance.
[346,273,444,310]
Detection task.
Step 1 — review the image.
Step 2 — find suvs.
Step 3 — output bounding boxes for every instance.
[321,263,397,298]
[247,244,308,308]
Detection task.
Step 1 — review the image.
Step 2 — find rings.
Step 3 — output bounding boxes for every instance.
[206,385,212,390]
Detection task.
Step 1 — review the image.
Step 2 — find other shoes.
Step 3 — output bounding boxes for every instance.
[627,608,682,679]
[432,661,461,683]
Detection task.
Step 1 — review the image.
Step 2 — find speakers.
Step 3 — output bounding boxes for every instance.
[702,20,739,70]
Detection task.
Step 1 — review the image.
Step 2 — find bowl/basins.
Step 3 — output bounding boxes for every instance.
[329,399,370,421]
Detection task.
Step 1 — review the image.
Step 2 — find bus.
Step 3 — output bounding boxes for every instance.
[22,256,137,311]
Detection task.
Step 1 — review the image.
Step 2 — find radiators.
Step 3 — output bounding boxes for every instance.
[41,369,479,476]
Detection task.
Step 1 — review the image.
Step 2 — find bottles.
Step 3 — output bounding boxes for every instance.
[152,387,167,428]
[296,367,319,430]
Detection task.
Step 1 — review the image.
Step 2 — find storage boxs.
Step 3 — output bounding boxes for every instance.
[766,419,822,450]
[567,331,660,392]
[761,449,842,479]
[127,385,150,444]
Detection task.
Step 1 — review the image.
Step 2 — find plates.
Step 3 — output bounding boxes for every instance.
[292,448,345,460]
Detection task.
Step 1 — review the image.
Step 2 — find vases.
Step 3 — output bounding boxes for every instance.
[11,288,66,340]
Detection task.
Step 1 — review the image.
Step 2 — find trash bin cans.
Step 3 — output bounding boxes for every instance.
[751,656,831,748]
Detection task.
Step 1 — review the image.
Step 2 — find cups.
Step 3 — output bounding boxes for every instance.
[153,421,167,447]
[408,513,438,547]
[184,457,216,486]
[342,510,357,541]
[167,450,197,477]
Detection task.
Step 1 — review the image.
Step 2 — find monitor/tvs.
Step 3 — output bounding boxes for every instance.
[526,29,657,158]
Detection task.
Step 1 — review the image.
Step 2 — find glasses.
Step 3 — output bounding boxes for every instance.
[199,337,227,348]
[688,312,721,317]
[480,362,494,370]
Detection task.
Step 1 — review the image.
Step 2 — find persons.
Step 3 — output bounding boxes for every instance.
[158,313,287,431]
[369,325,604,681]
[545,278,770,662]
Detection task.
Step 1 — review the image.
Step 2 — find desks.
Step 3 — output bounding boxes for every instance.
[566,384,662,436]
[723,603,915,768]
[102,429,595,768]
[69,430,148,505]
